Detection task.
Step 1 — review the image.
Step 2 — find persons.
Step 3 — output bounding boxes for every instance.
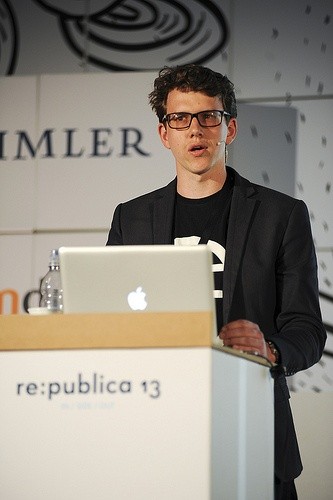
[105,64,328,500]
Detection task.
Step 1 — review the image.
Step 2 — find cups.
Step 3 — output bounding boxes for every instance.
[28,308,48,315]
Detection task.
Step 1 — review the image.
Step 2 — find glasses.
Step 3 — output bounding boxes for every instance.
[162,110,231,129]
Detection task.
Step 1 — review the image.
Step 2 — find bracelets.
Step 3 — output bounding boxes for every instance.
[268,341,280,362]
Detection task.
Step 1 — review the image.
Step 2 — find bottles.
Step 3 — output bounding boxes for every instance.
[40,250,62,314]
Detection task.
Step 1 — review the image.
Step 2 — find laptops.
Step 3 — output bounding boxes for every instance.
[57,244,225,348]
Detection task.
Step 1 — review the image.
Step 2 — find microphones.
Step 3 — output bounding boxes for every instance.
[216,141,225,146]
